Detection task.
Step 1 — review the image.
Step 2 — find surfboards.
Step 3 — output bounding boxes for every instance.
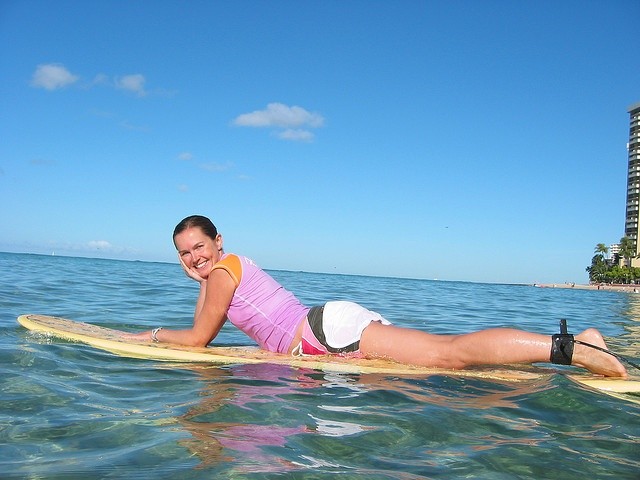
[17,314,640,393]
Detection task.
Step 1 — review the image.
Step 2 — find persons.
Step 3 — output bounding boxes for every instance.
[120,215,624,378]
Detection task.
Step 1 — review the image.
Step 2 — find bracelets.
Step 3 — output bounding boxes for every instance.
[150,327,163,342]
